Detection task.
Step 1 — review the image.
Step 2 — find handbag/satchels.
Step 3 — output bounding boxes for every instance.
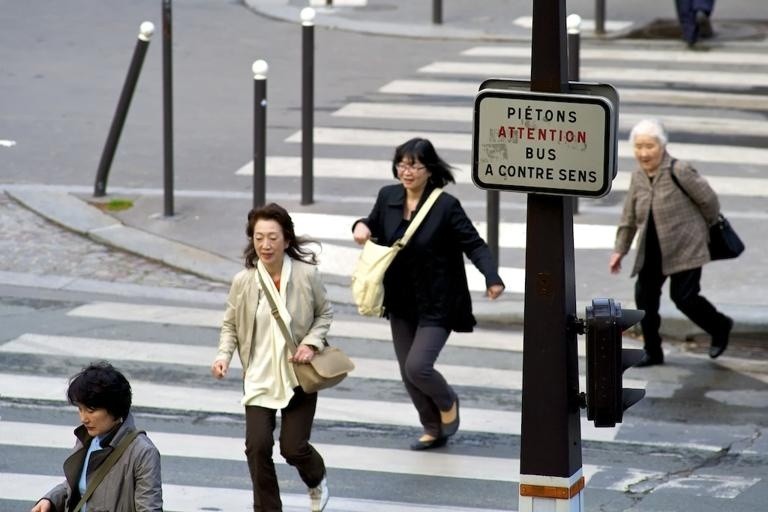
[290,345,354,392]
[350,235,403,319]
[707,212,745,261]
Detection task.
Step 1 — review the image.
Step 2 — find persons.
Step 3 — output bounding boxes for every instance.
[350,139,506,451]
[674,1,717,49]
[208,203,335,510]
[29,359,163,512]
[610,116,735,366]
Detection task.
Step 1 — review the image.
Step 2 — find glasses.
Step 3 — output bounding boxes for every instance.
[396,162,423,174]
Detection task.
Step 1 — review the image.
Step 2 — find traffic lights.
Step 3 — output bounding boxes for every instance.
[587,298,646,428]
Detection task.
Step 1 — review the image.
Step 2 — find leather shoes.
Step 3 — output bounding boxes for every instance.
[696,11,714,39]
[410,431,448,450]
[441,395,460,436]
[634,351,664,367]
[709,315,734,358]
[309,476,328,511]
